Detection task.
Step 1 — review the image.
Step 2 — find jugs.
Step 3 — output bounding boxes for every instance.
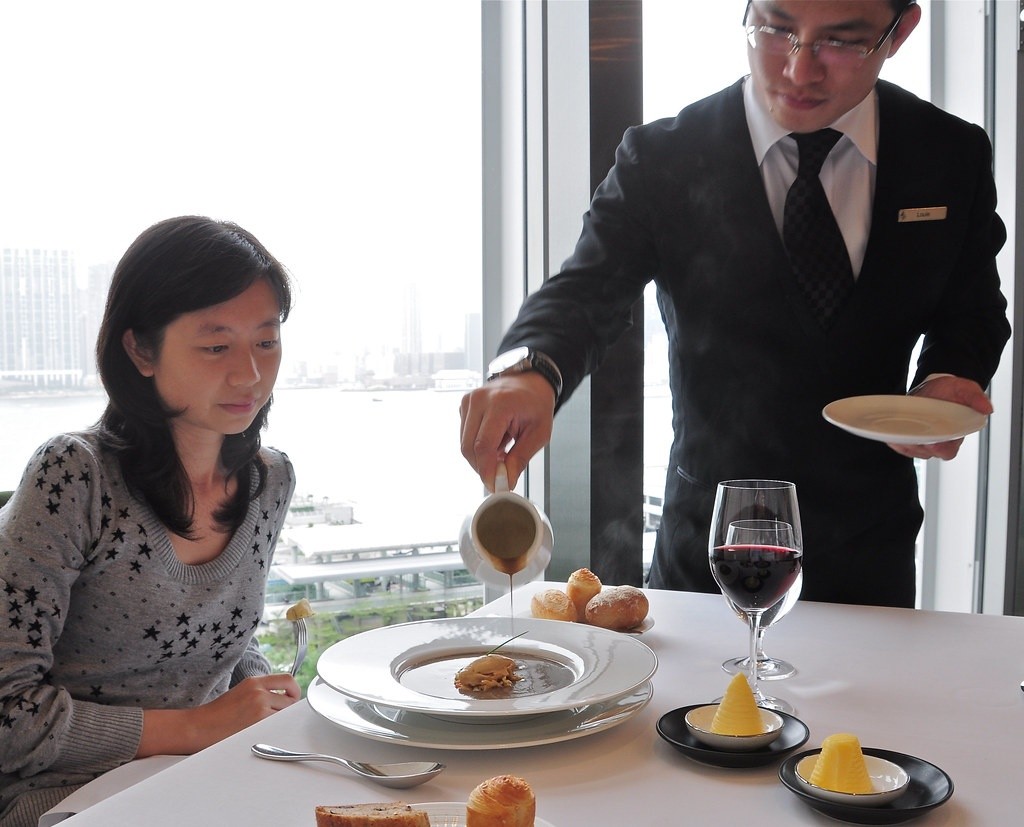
[456,459,552,590]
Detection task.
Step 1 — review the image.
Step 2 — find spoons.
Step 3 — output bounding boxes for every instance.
[251,743,447,790]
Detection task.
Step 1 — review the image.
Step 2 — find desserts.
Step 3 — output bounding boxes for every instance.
[709,673,765,735]
[809,734,875,795]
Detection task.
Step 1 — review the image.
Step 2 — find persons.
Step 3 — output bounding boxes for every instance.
[1,214,300,825]
[459,0,1011,611]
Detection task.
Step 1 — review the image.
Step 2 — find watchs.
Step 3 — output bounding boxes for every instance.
[485,346,562,403]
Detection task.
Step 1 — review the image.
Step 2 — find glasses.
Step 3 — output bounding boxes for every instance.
[742,0,915,68]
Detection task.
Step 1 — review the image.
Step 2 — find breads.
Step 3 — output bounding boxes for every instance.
[316,802,430,827]
[531,567,649,628]
[465,774,536,827]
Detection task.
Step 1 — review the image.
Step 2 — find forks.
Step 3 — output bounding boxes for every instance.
[287,617,308,677]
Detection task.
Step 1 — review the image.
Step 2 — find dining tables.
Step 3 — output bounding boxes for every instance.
[53,580,1024,827]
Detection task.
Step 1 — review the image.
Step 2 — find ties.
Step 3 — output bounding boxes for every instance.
[782,129,854,322]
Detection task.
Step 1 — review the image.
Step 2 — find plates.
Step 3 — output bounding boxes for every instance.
[777,747,954,825]
[684,704,785,752]
[306,675,654,751]
[315,616,658,718]
[821,393,990,446]
[793,752,911,807]
[402,801,558,827]
[655,704,810,767]
[503,605,655,636]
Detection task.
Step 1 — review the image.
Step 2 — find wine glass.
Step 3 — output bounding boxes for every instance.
[721,521,798,680]
[708,478,802,716]
